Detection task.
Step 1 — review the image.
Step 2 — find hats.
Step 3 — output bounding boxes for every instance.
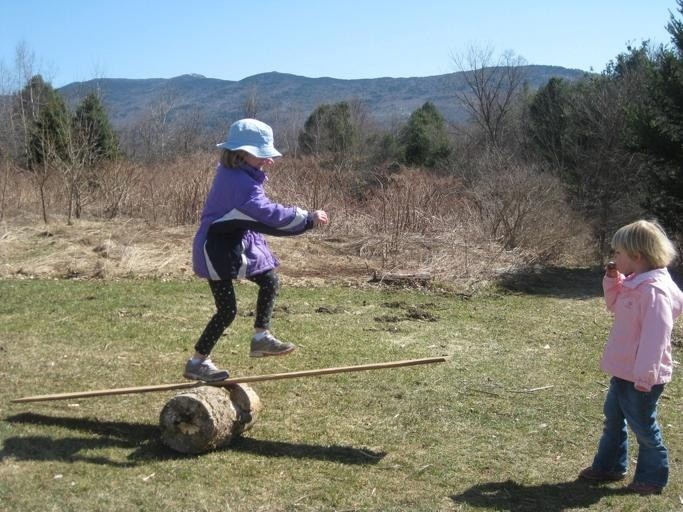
[215,117,283,158]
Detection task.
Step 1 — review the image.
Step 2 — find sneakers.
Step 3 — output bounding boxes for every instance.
[249,336,295,357]
[628,480,662,493]
[181,359,229,382]
[580,465,626,481]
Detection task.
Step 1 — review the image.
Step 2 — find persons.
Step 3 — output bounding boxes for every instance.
[574,217,683,498]
[179,116,330,383]
